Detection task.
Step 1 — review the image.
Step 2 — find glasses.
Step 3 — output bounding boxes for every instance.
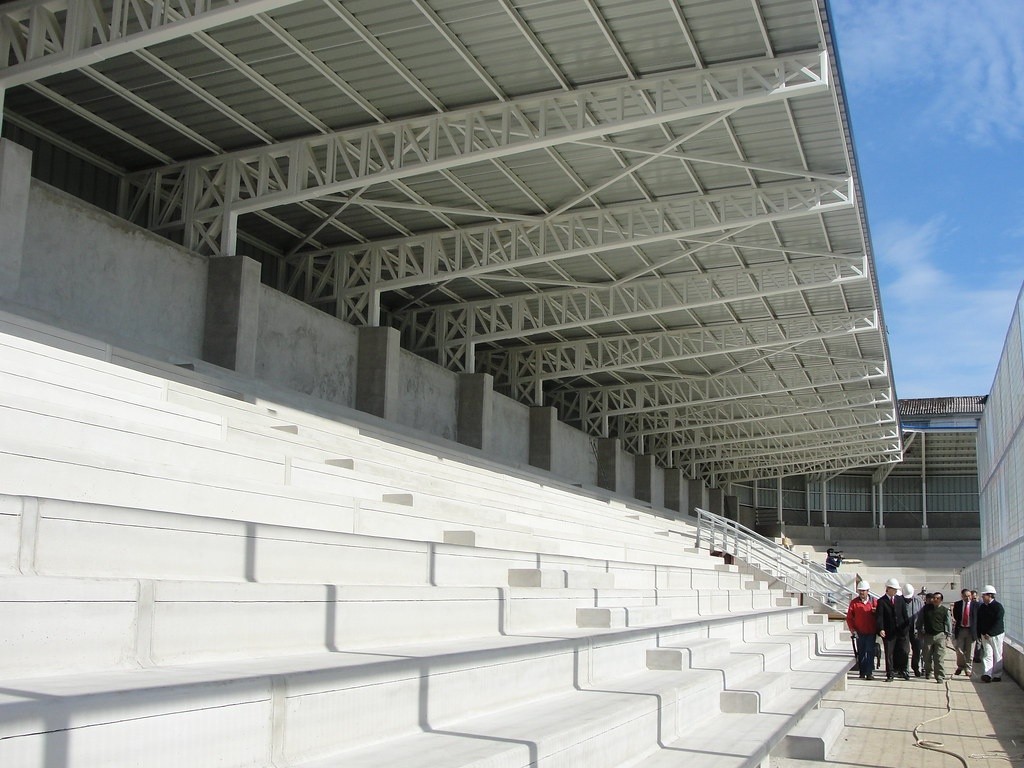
[933,597,941,600]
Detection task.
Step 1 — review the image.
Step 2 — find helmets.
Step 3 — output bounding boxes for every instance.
[857,580,871,590]
[885,578,901,589]
[980,585,997,594]
[902,583,915,598]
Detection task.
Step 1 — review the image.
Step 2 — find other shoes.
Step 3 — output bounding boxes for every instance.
[900,671,909,680]
[965,672,972,676]
[926,674,930,678]
[893,672,903,678]
[937,679,943,683]
[886,676,893,682]
[866,675,874,679]
[981,675,991,682]
[921,671,926,676]
[859,674,865,678]
[955,669,962,675]
[915,671,920,676]
[992,677,1001,681]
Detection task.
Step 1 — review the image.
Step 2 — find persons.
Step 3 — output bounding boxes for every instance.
[916,592,951,683]
[899,584,921,677]
[921,592,935,672]
[971,591,985,662]
[952,589,978,676]
[876,578,911,682]
[846,580,878,680]
[826,548,843,604]
[977,585,1005,682]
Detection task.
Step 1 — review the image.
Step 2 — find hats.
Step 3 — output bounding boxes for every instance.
[926,593,933,598]
[921,586,927,590]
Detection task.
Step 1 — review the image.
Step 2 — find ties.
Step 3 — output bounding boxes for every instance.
[891,596,894,604]
[963,602,968,626]
